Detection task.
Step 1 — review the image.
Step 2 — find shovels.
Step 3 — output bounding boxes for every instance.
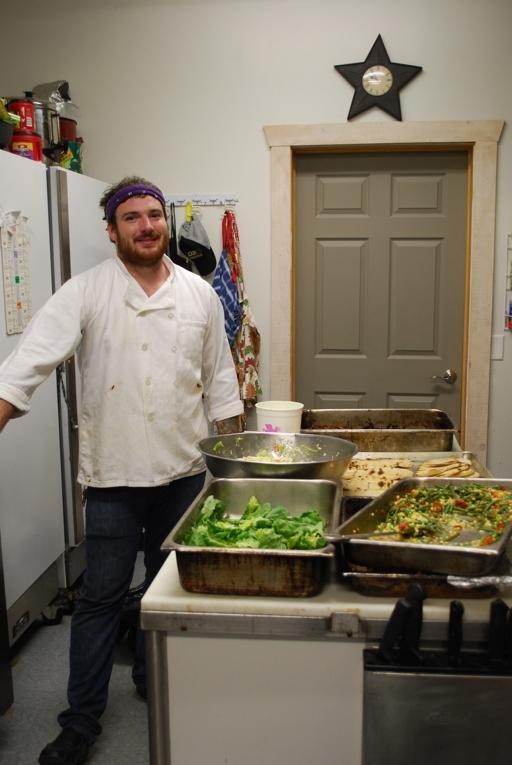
[342,457,480,493]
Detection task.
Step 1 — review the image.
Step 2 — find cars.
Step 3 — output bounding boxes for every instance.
[254,400,305,433]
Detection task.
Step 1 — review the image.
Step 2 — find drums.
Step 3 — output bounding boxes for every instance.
[376,583,512,675]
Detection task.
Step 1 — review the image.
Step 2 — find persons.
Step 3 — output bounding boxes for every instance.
[1,175,250,765]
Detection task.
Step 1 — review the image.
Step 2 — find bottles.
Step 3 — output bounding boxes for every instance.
[178,212,216,276]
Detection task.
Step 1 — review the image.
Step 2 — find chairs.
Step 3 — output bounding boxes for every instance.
[38,728,89,765]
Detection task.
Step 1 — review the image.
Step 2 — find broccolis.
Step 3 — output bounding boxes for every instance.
[0,149,119,648]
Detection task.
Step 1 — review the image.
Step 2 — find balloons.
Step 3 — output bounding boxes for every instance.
[333,33,422,121]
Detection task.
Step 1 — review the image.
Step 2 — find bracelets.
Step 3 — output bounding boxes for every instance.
[196,431,359,479]
